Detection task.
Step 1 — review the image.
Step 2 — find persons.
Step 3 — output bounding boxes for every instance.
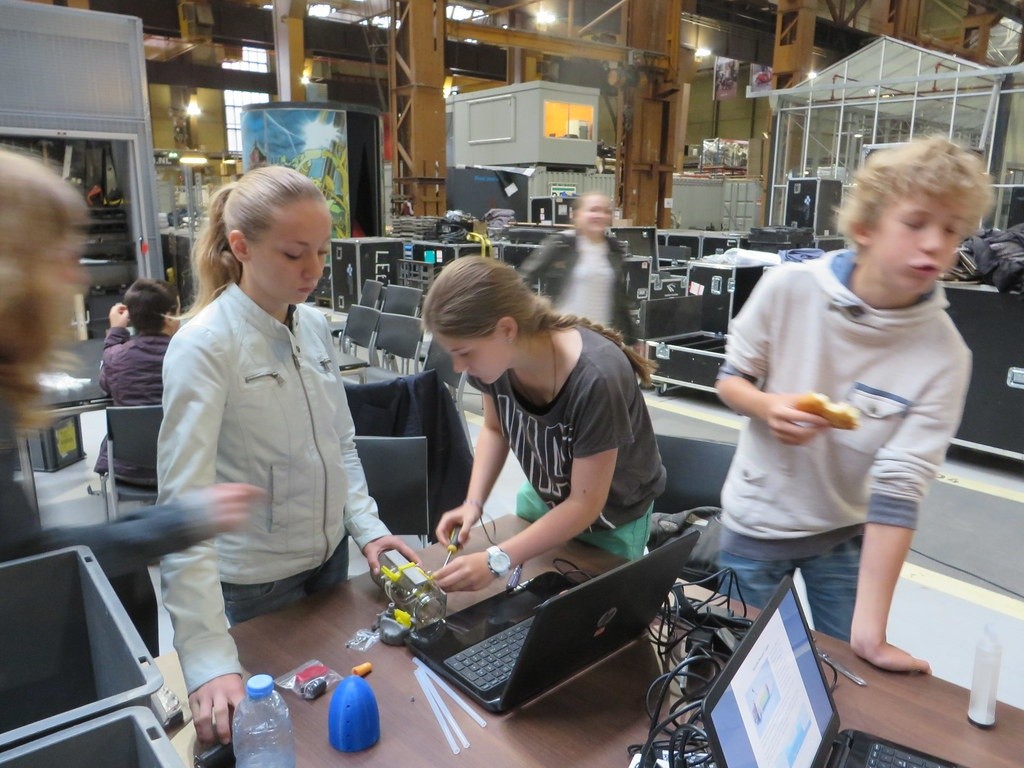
[716,135,994,679]
[519,192,642,353]
[93,277,181,489]
[153,164,425,744]
[0,148,266,583]
[422,254,667,593]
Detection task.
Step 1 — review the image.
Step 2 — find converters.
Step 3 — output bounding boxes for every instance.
[683,597,748,662]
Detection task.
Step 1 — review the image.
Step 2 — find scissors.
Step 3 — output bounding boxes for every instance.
[812,632,867,686]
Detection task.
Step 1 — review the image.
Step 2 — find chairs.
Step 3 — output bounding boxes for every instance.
[651,433,737,589]
[329,281,476,549]
[100,405,162,523]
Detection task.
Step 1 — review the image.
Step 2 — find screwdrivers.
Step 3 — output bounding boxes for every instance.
[442,523,461,568]
[506,564,524,590]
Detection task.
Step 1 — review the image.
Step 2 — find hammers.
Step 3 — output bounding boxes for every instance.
[36,139,54,160]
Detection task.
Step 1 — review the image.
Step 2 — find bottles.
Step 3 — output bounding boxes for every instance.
[232,673,295,768]
[371,549,445,626]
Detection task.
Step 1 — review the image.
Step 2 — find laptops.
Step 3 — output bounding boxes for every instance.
[404,530,702,714]
[701,573,969,768]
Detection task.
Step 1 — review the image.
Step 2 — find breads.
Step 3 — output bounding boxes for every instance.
[794,389,861,431]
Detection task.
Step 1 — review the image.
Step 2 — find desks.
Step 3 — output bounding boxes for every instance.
[141,514,1023,768]
[15,335,138,530]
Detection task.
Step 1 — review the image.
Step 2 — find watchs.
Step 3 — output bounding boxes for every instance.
[486,546,511,578]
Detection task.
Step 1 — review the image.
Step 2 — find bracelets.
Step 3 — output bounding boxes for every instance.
[463,501,484,515]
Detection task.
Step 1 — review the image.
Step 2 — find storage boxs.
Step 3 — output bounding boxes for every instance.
[315,177,1024,461]
[0,705,186,768]
[13,414,87,474]
[0,544,163,749]
